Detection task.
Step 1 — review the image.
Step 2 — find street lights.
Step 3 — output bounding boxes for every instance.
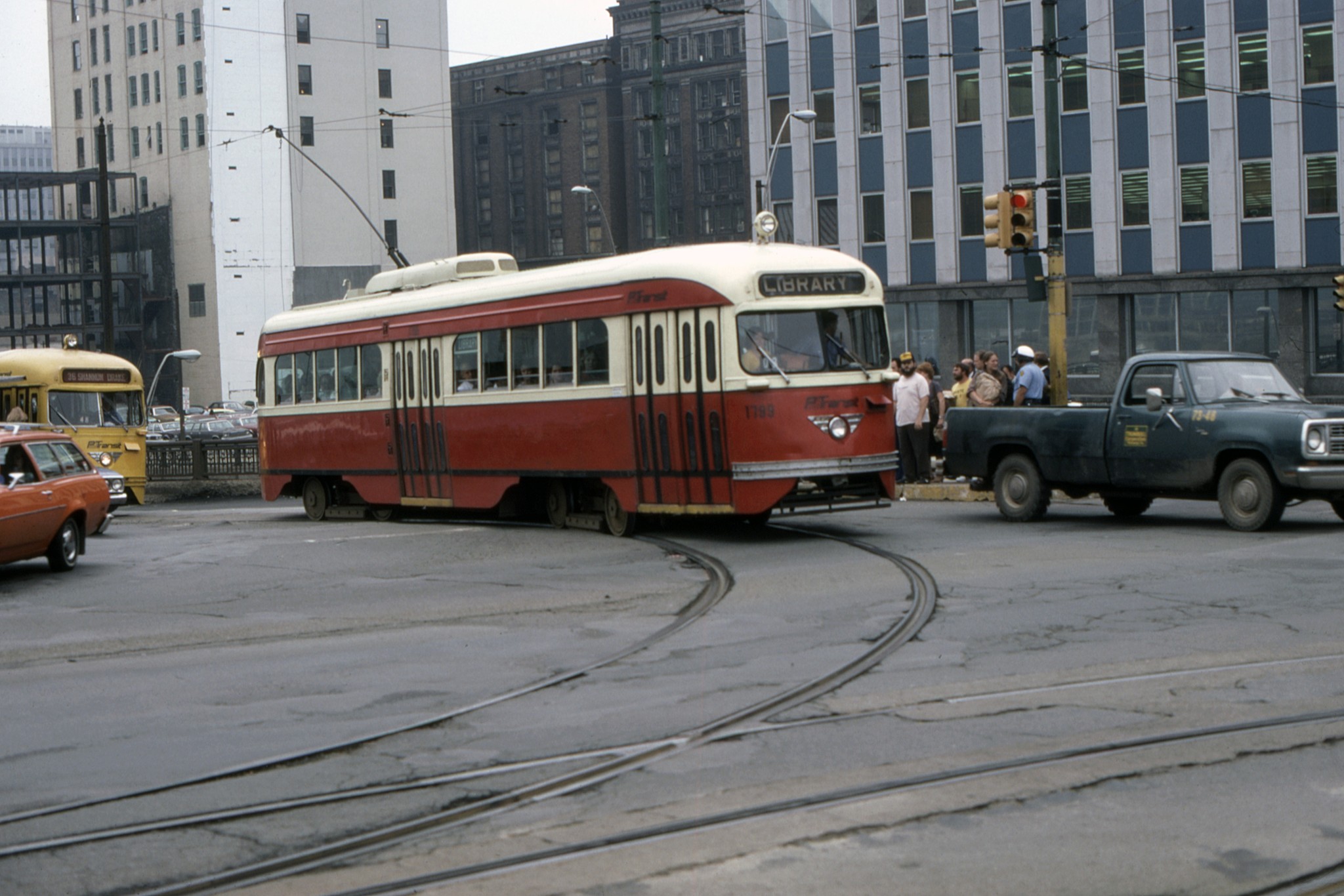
[570,185,618,255]
[762,108,819,208]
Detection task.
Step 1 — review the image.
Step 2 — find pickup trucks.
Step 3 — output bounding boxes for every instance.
[942,349,1344,535]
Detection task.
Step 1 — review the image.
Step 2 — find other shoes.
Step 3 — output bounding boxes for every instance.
[943,477,956,483]
[956,476,966,482]
[914,478,930,485]
[897,476,914,484]
[971,477,977,481]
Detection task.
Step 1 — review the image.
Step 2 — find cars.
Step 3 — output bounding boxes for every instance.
[149,396,264,440]
[0,422,128,573]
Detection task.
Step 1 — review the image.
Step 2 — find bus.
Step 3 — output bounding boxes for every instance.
[249,205,901,538]
[0,334,149,506]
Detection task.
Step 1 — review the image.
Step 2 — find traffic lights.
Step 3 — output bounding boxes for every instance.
[984,190,1013,250]
[1012,190,1035,250]
[1332,274,1344,311]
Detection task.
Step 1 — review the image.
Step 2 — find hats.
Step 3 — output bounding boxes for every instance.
[900,351,914,363]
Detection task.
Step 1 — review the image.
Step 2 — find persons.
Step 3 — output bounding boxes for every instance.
[277,347,600,403]
[741,328,773,373]
[891,344,1051,485]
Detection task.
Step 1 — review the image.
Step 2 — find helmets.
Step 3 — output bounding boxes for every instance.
[1011,346,1034,362]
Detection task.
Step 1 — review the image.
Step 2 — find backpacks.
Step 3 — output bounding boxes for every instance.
[970,373,1002,407]
